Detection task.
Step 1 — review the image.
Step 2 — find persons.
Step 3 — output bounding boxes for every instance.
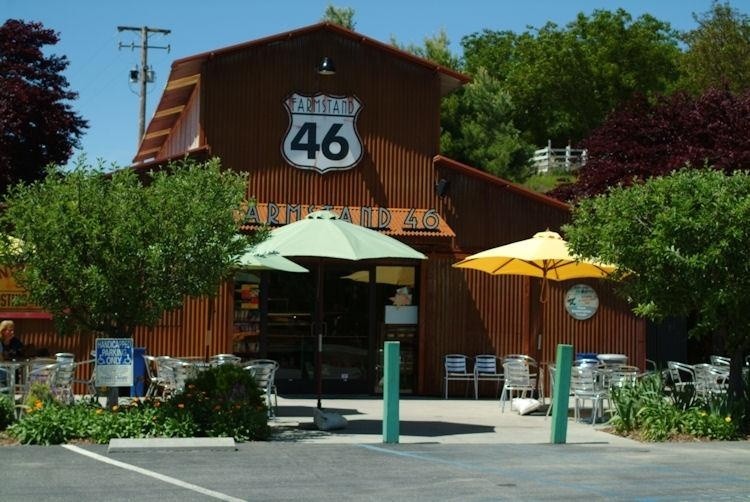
[0,317,25,392]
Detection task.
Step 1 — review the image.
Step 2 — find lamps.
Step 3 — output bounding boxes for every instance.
[313,55,338,76]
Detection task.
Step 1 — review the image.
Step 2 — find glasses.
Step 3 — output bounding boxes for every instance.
[5,330,14,334]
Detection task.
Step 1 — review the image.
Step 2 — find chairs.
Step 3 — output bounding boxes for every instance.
[440,354,473,399]
[143,352,279,423]
[0,349,102,416]
[501,354,749,427]
[473,354,500,400]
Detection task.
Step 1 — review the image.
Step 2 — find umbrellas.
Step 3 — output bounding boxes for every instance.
[0,230,36,256]
[220,233,310,277]
[252,208,431,412]
[450,223,639,400]
